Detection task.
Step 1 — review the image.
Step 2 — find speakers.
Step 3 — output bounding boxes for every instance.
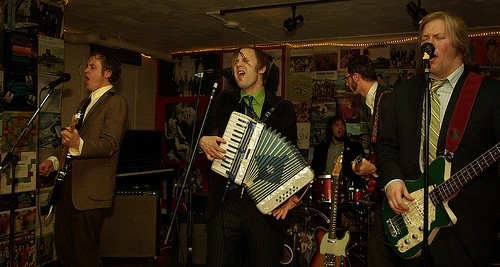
[97,190,157,257]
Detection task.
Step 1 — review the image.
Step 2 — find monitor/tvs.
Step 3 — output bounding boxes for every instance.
[116,130,164,174]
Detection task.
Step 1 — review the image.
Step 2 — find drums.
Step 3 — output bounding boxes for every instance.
[316,175,350,203]
[353,190,362,201]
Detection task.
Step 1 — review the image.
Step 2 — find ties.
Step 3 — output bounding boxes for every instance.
[421,78,448,172]
[77,96,91,129]
[246,96,255,117]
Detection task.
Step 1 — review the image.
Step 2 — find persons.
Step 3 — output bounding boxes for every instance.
[38,48,129,267]
[379,11,500,267]
[345,54,418,267]
[310,116,365,214]
[196,44,300,265]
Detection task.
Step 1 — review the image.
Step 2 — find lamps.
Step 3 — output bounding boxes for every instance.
[405,1,428,23]
[282,6,304,33]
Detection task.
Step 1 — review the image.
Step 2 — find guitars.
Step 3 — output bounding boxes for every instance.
[47,111,82,207]
[382,143,500,259]
[309,155,350,267]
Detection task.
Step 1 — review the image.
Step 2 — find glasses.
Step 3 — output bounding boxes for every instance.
[342,73,355,82]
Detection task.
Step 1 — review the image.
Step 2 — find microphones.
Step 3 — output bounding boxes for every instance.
[419,40,435,73]
[348,133,365,141]
[41,73,71,91]
[217,67,233,79]
